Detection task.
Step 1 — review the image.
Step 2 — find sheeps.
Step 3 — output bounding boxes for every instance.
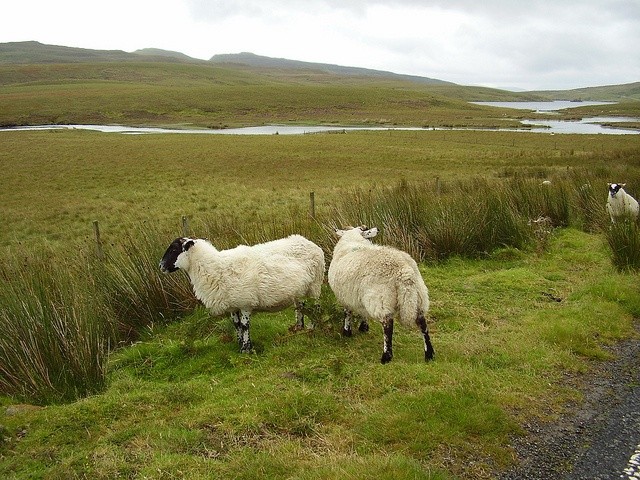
[328,223,433,364]
[158,234,326,355]
[605,182,640,230]
